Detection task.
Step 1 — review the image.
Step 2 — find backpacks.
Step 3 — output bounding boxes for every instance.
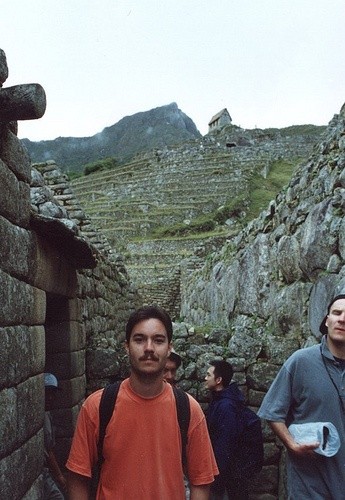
[210,397,264,479]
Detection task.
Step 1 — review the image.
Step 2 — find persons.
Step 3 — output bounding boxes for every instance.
[64,302,218,500]
[202,355,266,499]
[258,296,344,499]
[43,372,69,500]
[157,349,181,389]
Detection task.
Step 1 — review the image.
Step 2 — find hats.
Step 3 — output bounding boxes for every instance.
[45,373,63,391]
[169,352,181,369]
[318,294,344,334]
